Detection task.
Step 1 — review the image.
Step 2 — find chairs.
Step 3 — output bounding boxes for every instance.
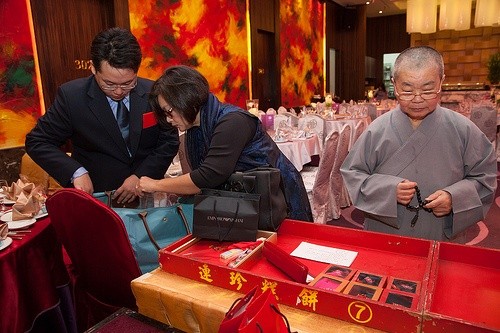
[46,188,146,317]
[245,95,500,225]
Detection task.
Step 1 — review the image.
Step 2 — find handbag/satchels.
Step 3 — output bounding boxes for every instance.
[223,167,288,230]
[92,189,194,274]
[192,189,261,243]
[218,286,298,333]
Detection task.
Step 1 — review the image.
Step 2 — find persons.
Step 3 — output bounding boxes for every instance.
[25,27,180,198]
[135,65,312,222]
[339,47,496,246]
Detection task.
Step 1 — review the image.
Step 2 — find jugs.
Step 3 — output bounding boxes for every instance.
[274,130,288,140]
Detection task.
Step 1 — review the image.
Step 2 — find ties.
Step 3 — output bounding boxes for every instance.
[116,97,132,157]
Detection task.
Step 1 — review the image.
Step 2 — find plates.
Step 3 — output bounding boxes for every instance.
[274,141,286,143]
[0,187,50,251]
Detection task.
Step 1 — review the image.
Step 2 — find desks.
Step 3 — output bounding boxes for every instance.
[376,106,395,116]
[469,102,500,125]
[266,128,319,171]
[0,186,71,333]
[359,103,379,120]
[320,113,371,147]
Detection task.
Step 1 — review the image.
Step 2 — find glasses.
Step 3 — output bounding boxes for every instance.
[394,81,442,101]
[97,73,137,90]
[163,107,175,119]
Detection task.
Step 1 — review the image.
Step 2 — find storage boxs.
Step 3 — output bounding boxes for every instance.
[156,224,435,333]
[422,242,500,333]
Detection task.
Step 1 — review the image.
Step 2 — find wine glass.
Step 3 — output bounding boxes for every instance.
[0,179,10,211]
